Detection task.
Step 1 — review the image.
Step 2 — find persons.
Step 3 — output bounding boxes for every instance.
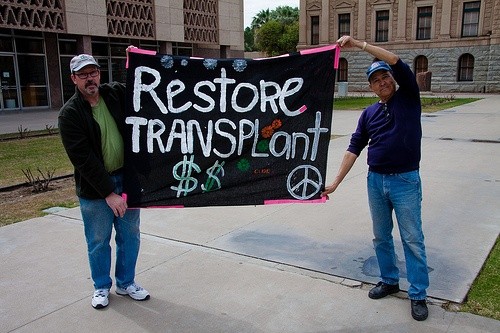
[58,45,150,310]
[321,34,430,321]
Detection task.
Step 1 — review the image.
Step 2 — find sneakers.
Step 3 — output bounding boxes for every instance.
[368,281,399,299]
[91,288,110,308]
[411,299,428,321]
[115,282,150,301]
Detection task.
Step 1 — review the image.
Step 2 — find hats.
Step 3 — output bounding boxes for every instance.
[70,54,100,73]
[366,61,392,78]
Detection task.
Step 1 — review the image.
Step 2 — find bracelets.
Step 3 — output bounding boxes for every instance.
[362,42,367,50]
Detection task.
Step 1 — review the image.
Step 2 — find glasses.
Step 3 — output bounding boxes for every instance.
[74,67,99,79]
